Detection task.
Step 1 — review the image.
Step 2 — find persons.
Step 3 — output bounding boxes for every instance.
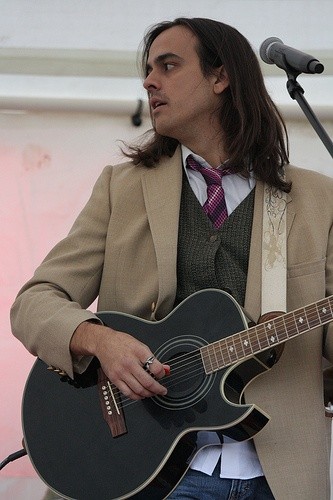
[10,18,333,500]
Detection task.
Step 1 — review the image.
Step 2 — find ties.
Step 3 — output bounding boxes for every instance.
[186,154,238,228]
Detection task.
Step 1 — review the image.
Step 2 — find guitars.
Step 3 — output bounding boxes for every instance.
[20,287,333,500]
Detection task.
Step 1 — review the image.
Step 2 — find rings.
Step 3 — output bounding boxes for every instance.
[143,355,155,369]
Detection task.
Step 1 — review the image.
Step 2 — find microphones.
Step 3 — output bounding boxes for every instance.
[260,37,324,74]
